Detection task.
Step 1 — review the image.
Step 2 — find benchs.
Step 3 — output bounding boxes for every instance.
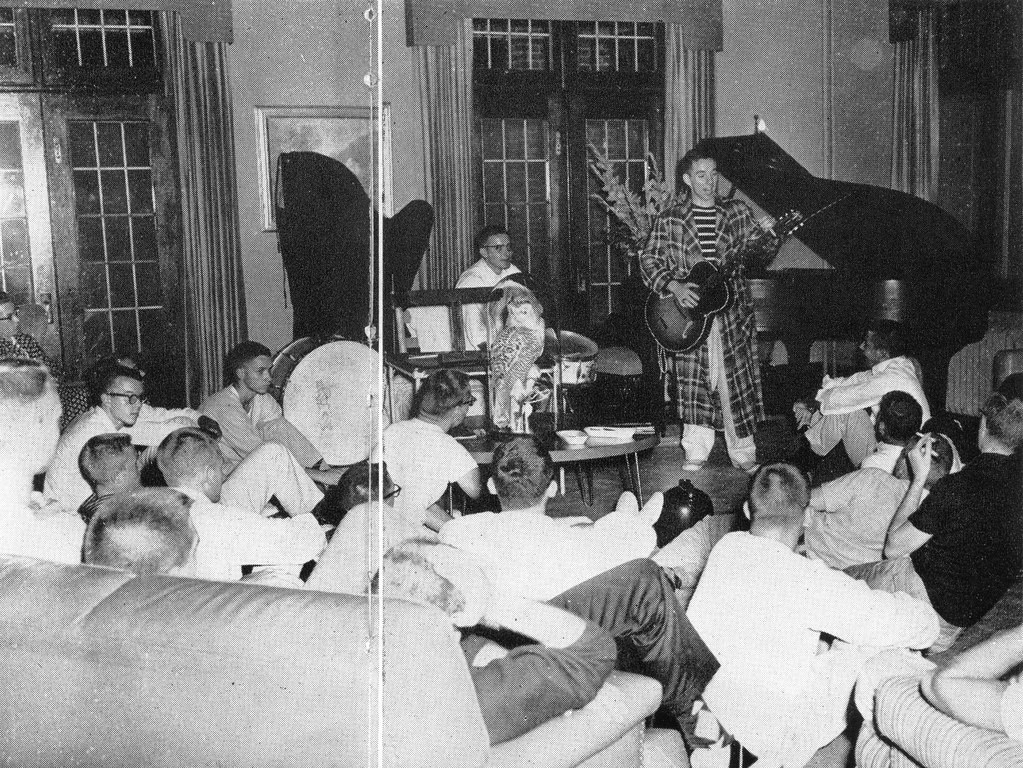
[385,288,559,432]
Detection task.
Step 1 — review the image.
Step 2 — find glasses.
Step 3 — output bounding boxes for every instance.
[0,307,20,321]
[484,243,511,251]
[385,485,400,498]
[465,396,476,406]
[109,393,148,405]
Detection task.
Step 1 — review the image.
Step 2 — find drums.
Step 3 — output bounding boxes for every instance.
[536,327,602,390]
[268,332,389,468]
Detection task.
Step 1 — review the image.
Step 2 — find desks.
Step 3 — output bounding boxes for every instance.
[445,413,661,518]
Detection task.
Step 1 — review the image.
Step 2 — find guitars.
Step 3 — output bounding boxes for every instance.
[644,209,806,353]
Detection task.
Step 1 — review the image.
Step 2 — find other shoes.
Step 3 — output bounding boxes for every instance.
[617,491,638,515]
[683,459,705,472]
[741,462,760,477]
[638,492,664,528]
[793,403,812,427]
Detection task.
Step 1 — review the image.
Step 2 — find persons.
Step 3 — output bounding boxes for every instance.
[638,147,778,475]
[796,317,935,469]
[453,226,541,353]
[0,295,1023,767]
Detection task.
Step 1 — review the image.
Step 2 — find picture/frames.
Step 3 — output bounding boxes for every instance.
[254,104,393,233]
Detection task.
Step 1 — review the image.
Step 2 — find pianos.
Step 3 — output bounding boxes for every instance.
[701,131,988,402]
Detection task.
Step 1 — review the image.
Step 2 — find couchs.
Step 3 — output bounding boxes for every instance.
[856,674,1023,768]
[0,552,692,768]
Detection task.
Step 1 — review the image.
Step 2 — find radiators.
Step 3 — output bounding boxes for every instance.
[946,310,1023,416]
